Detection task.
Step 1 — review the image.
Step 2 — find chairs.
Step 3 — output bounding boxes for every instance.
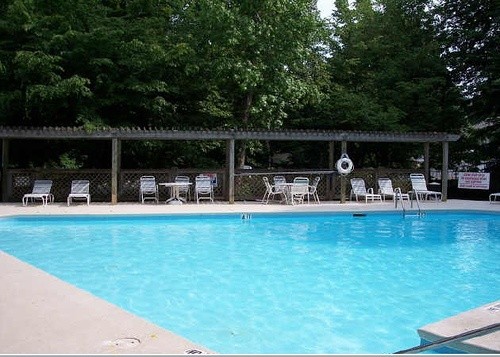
[407,173,442,202]
[173,176,190,204]
[378,178,409,203]
[22,180,54,207]
[350,178,381,202]
[261,176,321,205]
[140,176,158,204]
[195,176,214,203]
[488,193,500,207]
[67,180,91,206]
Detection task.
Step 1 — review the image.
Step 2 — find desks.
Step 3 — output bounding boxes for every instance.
[279,183,301,206]
[159,183,193,205]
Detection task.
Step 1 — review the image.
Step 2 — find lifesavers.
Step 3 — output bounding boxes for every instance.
[336,158,353,174]
